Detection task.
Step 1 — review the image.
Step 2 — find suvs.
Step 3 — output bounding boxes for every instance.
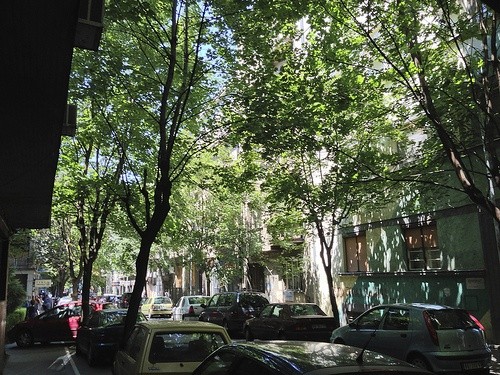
[199,291,272,340]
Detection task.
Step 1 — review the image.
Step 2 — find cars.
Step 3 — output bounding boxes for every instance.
[329,303,493,375]
[112,320,232,375]
[12,300,118,350]
[242,302,338,343]
[191,340,436,375]
[75,311,148,368]
[43,292,133,310]
[170,295,215,322]
[141,296,175,319]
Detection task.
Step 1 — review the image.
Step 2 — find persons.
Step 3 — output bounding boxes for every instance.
[24,300,39,320]
[39,290,52,310]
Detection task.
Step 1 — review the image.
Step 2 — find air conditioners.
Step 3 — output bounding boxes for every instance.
[62,0,105,137]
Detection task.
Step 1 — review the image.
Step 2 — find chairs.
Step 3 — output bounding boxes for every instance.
[189,340,208,353]
[387,318,400,330]
[151,337,165,353]
[120,316,128,325]
